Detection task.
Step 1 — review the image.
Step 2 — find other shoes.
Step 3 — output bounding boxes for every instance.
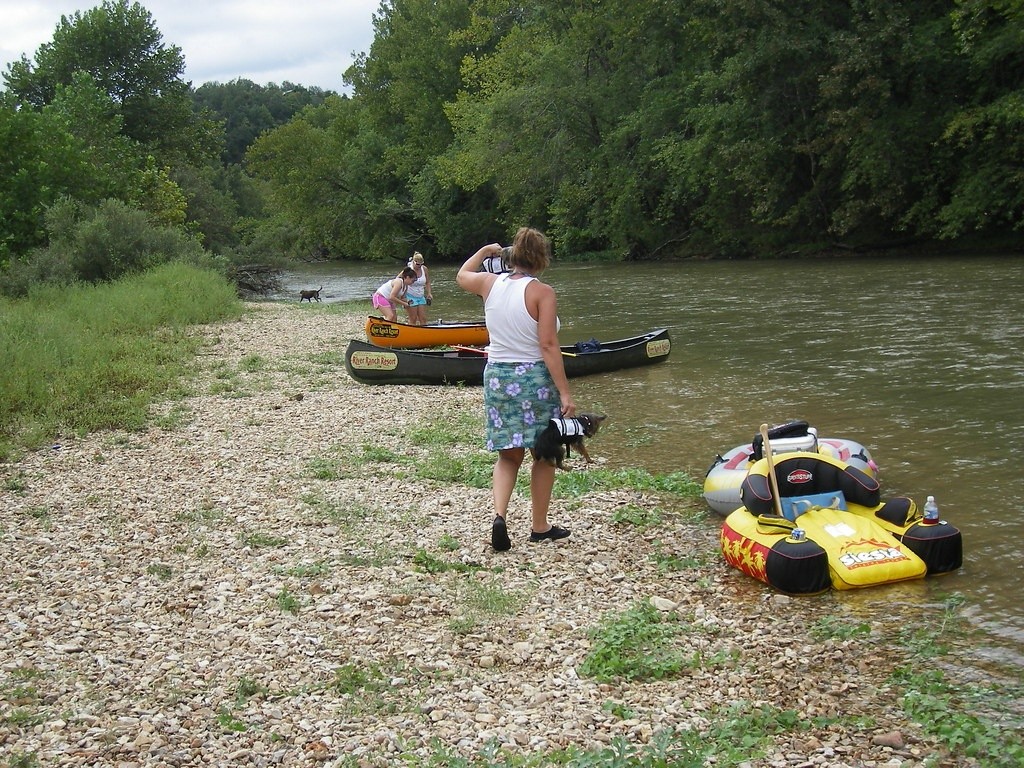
[492,513,511,551]
[530,525,570,542]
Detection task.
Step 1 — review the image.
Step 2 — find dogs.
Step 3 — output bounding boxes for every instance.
[299,285,323,303]
[533,414,609,471]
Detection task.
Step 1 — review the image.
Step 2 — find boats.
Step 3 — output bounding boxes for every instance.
[367,316,490,349]
[709,438,876,518]
[344,327,672,386]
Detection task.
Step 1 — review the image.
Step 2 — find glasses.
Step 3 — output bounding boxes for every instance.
[413,260,424,265]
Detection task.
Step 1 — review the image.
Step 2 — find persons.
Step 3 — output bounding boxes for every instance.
[456,226,577,551]
[372,267,417,323]
[396,250,433,325]
